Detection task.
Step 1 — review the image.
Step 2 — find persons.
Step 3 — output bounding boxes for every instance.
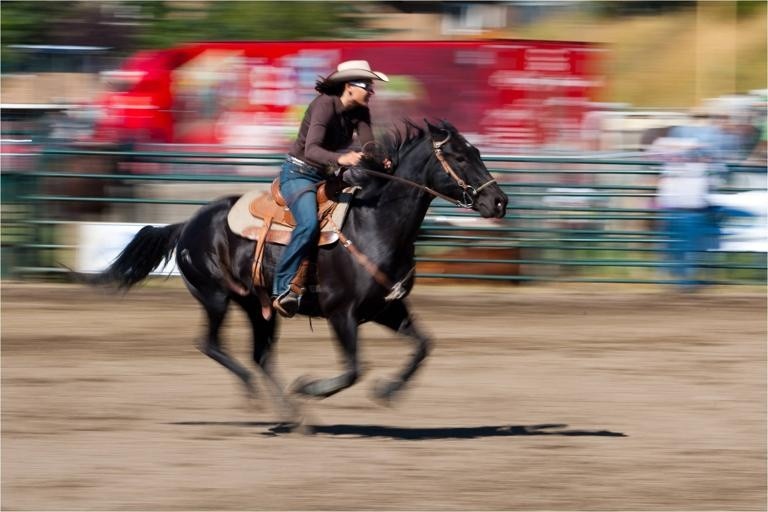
[272,58,393,308]
[647,85,767,293]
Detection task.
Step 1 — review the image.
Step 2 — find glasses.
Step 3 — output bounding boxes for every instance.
[349,81,372,89]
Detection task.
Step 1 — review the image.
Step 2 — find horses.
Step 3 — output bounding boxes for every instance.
[58,116,508,435]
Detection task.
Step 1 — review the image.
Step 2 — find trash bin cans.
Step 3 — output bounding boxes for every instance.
[660,203,727,291]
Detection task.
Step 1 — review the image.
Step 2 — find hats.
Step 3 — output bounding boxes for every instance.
[329,60,389,82]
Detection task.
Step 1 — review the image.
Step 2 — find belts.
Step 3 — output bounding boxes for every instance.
[292,157,316,170]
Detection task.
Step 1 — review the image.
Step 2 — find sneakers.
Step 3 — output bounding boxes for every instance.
[279,296,297,317]
